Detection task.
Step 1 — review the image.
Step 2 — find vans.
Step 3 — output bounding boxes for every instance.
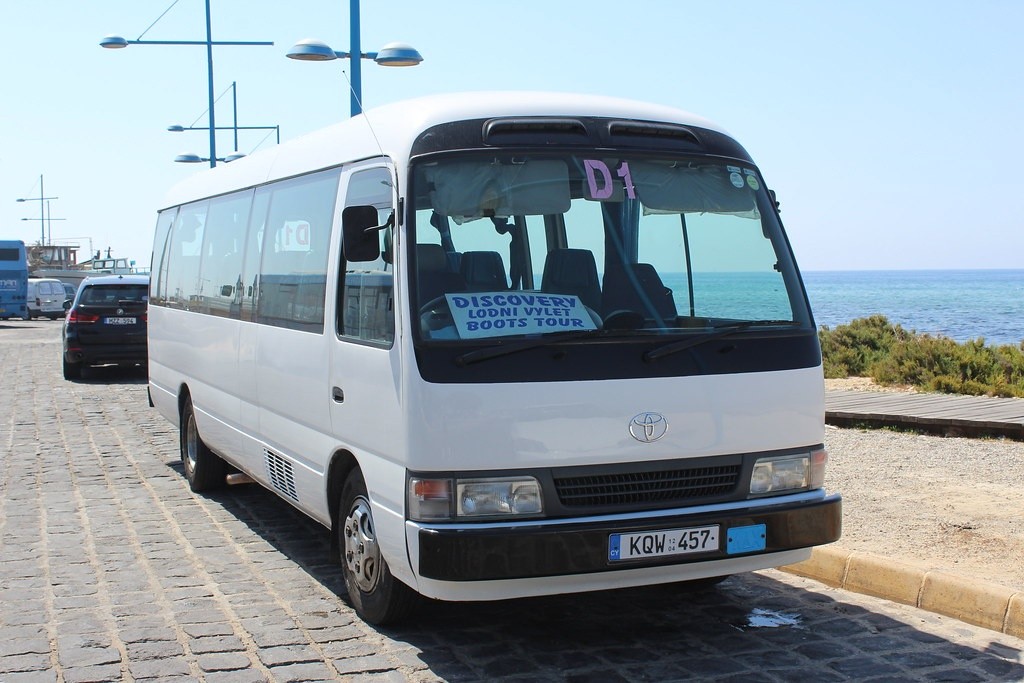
[27,278,77,320]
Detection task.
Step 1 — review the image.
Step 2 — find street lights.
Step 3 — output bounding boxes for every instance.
[286,1,422,118]
[15,175,67,246]
[98,0,280,169]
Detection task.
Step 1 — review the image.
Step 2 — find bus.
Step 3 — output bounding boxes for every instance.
[145,93,842,627]
[0,240,28,321]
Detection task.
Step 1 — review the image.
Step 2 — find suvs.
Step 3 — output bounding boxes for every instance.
[61,273,151,381]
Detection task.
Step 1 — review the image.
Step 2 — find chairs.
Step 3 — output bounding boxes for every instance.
[460,250,509,290]
[139,288,147,300]
[417,268,449,308]
[540,247,603,314]
[416,242,454,280]
[90,288,106,305]
[603,262,678,323]
[176,249,328,321]
